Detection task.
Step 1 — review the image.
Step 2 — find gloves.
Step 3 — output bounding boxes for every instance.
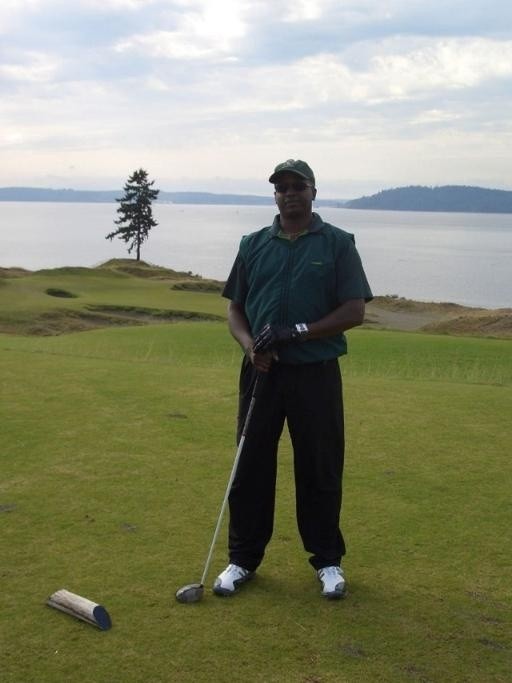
[253,323,301,354]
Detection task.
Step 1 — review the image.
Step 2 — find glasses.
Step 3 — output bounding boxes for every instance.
[275,183,312,192]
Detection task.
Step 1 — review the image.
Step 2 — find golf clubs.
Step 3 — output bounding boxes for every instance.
[176,369,262,604]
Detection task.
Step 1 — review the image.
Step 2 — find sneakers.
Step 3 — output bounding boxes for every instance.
[318,565,346,599]
[213,564,256,596]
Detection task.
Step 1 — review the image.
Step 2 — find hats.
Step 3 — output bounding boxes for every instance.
[270,159,313,183]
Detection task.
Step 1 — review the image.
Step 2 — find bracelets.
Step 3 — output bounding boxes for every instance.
[294,321,309,337]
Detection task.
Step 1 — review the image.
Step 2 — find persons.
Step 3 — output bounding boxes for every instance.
[211,159,376,597]
